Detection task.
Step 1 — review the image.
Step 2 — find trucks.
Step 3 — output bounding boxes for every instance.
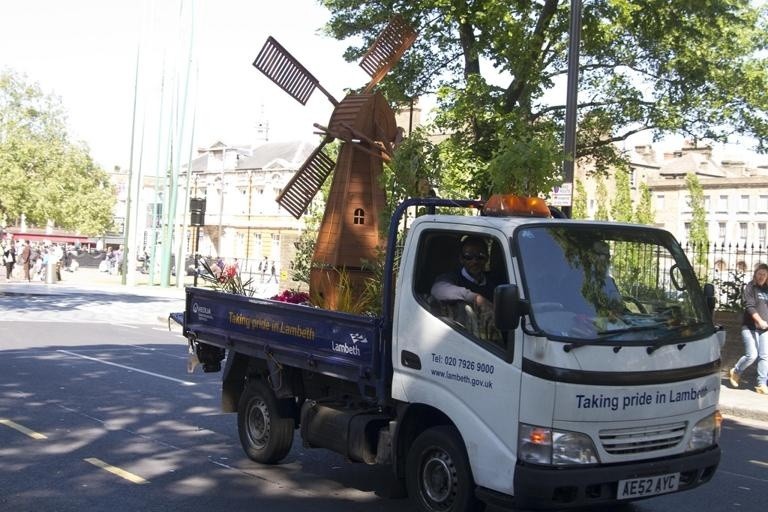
[168,193,725,511]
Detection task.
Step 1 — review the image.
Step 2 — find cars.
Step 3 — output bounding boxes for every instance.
[172,256,224,278]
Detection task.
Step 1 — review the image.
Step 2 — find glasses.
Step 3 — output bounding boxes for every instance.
[460,247,487,260]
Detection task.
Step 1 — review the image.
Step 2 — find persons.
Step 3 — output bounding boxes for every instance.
[729,263,768,395]
[0,240,279,285]
[430,237,499,313]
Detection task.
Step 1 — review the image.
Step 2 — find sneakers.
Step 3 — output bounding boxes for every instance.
[754,384,768,395]
[729,367,741,388]
